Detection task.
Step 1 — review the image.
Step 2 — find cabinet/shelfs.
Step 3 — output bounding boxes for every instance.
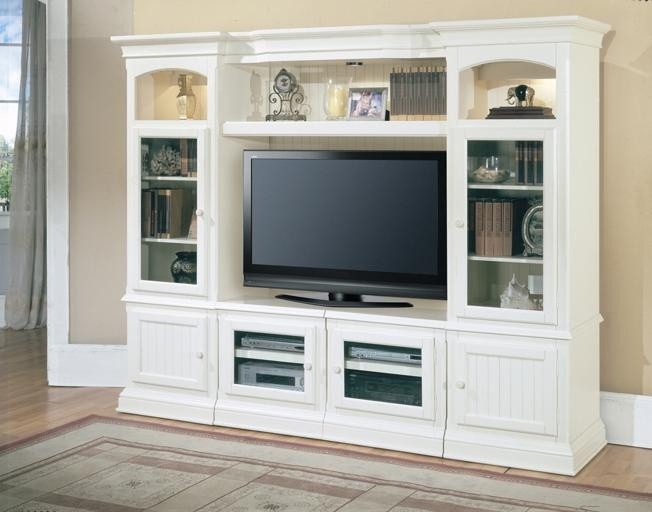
[107,16,609,478]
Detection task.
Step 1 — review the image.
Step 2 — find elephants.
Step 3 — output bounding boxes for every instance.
[504,86,534,108]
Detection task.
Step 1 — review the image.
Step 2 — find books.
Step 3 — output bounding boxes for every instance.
[467,139,542,258]
[390,61,446,123]
[139,135,198,240]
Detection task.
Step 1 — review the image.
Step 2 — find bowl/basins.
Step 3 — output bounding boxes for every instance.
[471,153,511,182]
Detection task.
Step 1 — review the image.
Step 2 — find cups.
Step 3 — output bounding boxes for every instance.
[324,75,351,119]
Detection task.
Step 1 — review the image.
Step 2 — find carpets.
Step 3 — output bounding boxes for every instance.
[0,413,652,512]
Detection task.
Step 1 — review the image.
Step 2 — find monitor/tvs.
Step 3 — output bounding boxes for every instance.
[243,148,446,307]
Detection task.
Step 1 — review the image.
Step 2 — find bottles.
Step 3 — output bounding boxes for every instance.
[177,74,196,119]
